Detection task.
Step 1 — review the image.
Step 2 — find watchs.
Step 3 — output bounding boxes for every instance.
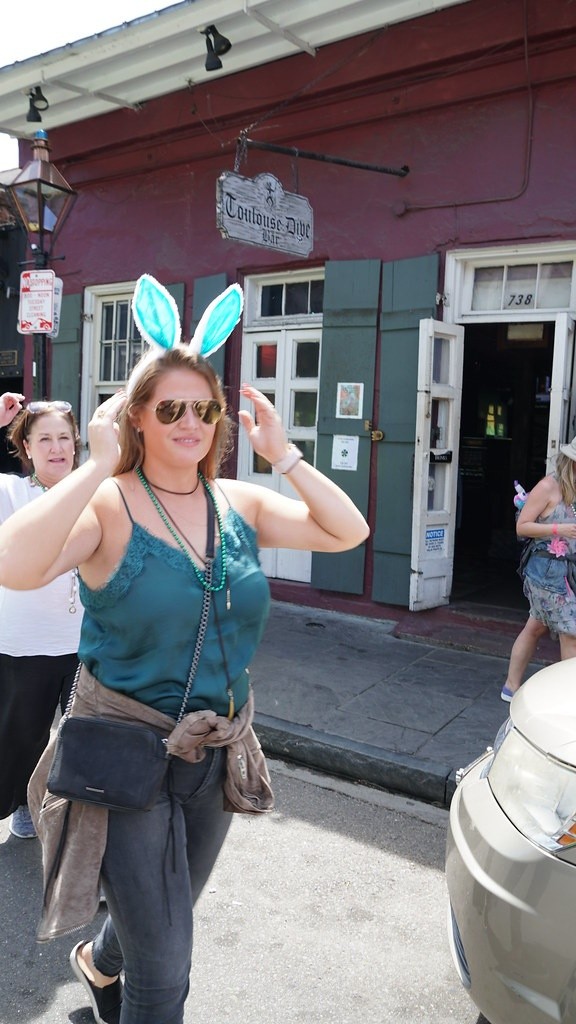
[273,444,304,474]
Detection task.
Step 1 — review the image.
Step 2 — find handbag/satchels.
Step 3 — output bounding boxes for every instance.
[48,714,170,812]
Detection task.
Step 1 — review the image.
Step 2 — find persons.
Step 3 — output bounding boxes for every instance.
[0,347,375,1023]
[499,437,576,701]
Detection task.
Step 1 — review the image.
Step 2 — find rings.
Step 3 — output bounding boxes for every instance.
[97,410,104,416]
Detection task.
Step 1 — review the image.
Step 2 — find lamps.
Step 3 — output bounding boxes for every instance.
[26,86,48,122]
[200,24,232,71]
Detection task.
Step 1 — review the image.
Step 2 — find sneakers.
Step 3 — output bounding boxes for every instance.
[9,804,38,838]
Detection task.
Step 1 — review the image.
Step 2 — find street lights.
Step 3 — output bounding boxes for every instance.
[7,128,79,401]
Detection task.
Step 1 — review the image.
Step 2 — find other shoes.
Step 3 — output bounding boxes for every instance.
[501,688,514,701]
[70,937,123,1024]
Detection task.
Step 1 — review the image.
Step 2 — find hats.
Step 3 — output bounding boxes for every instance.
[560,434,576,464]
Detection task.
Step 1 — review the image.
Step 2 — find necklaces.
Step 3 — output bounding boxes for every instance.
[31,472,77,616]
[134,467,233,611]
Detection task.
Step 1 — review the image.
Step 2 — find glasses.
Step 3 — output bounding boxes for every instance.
[20,400,73,422]
[142,399,224,425]
